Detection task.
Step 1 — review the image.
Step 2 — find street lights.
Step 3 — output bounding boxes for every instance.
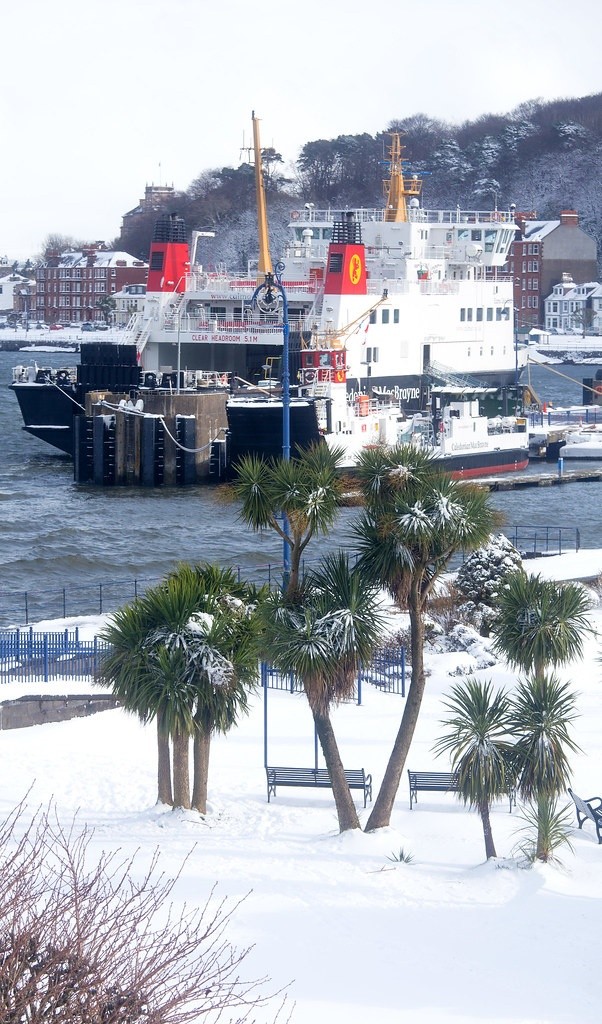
[246,259,294,596]
[500,299,520,416]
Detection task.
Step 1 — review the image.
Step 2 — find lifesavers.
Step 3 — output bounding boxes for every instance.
[290,210,301,220]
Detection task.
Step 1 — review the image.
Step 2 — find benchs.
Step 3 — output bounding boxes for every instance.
[407,769,517,814]
[568,788,602,844]
[265,765,373,808]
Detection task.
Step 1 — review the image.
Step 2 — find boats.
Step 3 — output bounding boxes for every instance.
[7,109,602,488]
